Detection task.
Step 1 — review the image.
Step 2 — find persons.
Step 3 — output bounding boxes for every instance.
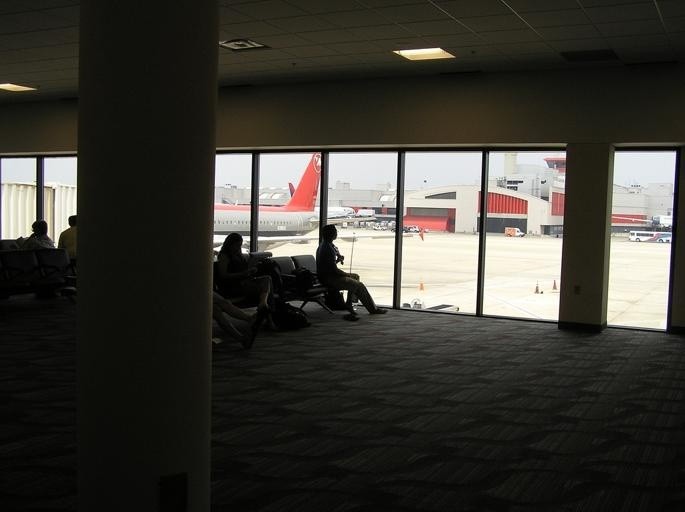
[57,215,77,270]
[316,224,388,316]
[213,290,257,348]
[216,233,279,332]
[16,220,54,249]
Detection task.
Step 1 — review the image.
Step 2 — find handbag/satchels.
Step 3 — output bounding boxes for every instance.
[271,298,312,332]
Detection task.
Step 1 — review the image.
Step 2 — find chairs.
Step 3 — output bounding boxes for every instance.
[212,253,340,321]
[0,236,78,306]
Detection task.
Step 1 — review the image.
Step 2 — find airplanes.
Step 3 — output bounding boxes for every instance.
[214,153,377,237]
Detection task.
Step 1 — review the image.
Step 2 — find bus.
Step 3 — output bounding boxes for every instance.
[629,231,672,243]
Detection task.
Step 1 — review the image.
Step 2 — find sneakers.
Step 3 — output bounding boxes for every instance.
[372,308,388,314]
[345,303,359,317]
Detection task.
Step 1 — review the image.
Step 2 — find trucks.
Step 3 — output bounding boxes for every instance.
[504,227,525,237]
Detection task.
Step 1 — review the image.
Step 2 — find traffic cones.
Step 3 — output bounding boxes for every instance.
[551,280,559,291]
[534,280,544,294]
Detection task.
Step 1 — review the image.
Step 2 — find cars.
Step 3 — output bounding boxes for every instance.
[373,221,419,233]
